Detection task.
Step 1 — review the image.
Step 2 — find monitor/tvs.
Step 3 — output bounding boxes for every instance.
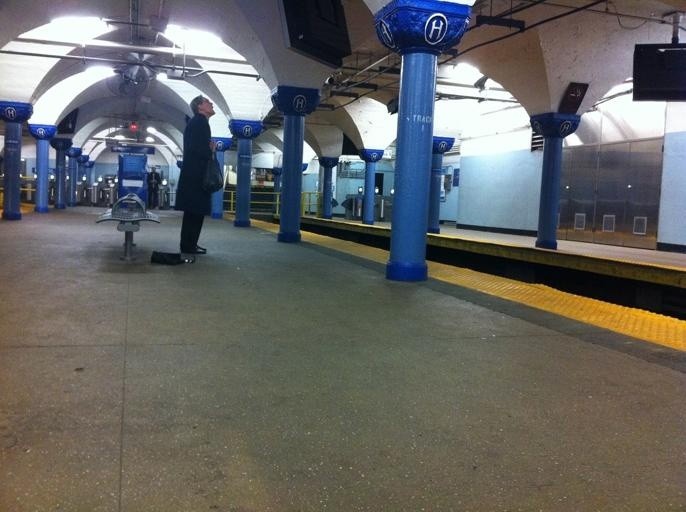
[277,0,352,70]
[632,43,685,102]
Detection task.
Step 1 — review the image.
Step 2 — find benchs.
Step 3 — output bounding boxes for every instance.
[96,192,160,260]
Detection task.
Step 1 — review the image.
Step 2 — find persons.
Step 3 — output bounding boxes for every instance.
[173,95,220,252]
[147,167,161,210]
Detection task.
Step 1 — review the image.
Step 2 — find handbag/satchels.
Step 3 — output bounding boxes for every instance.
[203,158,223,193]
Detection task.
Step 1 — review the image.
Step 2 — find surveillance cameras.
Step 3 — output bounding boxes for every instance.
[325,76,336,87]
[167,68,186,80]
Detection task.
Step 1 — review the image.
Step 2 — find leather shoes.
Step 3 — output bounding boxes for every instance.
[180,246,207,254]
